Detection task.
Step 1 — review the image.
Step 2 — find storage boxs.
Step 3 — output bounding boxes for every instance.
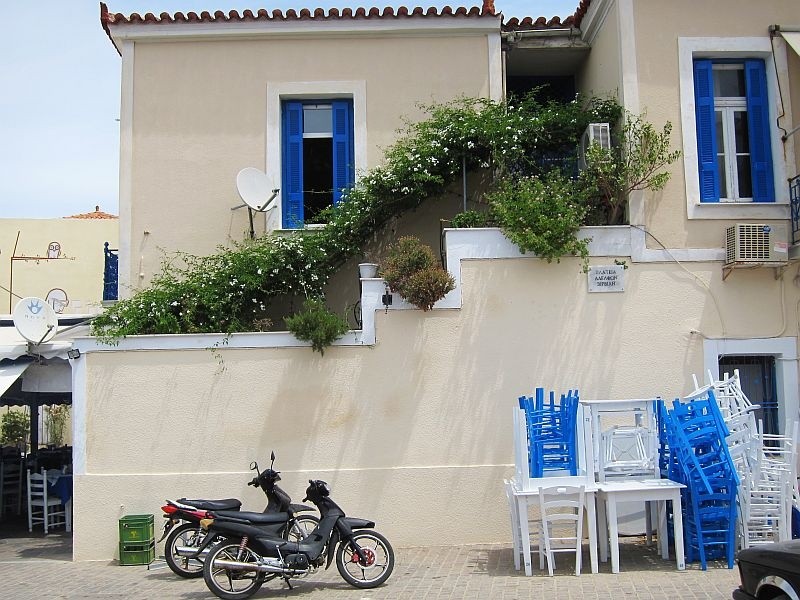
[119,515,156,566]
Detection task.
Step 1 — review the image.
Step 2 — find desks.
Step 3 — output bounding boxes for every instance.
[516,488,598,577]
[578,398,660,483]
[596,479,688,574]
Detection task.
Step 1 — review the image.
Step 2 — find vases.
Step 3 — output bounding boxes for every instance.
[359,262,380,278]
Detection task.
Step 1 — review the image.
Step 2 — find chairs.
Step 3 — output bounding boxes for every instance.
[0,445,73,533]
[503,368,800,576]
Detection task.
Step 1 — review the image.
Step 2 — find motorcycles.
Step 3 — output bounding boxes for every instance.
[157,450,331,579]
[193,480,394,600]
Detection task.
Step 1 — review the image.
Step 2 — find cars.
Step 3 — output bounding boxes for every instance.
[733,538,800,600]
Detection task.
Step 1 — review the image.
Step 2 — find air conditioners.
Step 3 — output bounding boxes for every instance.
[722,223,789,281]
[576,122,612,176]
